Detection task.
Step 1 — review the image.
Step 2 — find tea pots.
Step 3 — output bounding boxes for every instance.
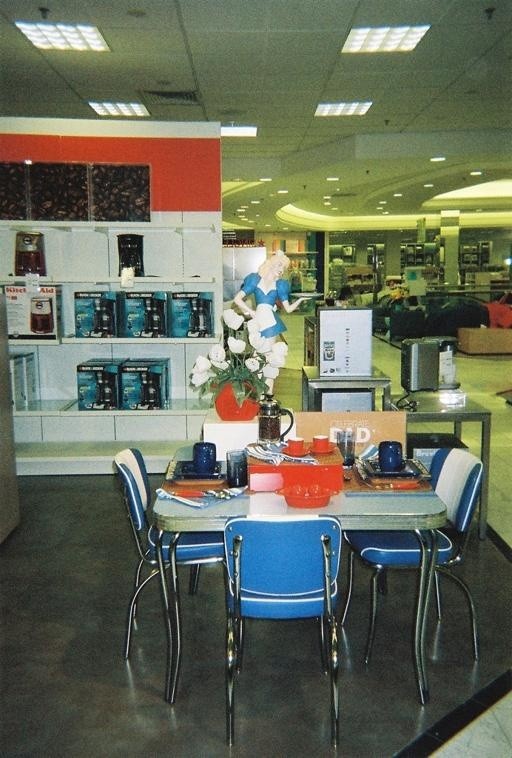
[259,393,295,443]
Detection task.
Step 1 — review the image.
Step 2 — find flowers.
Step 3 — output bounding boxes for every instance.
[191,303,290,406]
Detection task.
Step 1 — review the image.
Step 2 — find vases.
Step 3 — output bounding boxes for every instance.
[211,382,260,422]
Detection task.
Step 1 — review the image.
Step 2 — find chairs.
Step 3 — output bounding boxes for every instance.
[341,448,484,664]
[222,513,342,750]
[114,448,224,660]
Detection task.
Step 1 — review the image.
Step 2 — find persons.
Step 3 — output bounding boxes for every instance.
[233,249,313,352]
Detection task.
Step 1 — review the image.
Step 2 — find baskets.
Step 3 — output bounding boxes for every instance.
[279,486,338,508]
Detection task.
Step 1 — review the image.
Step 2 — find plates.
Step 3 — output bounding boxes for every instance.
[362,455,433,478]
[164,460,230,484]
[290,292,324,299]
[307,447,336,456]
[282,450,309,457]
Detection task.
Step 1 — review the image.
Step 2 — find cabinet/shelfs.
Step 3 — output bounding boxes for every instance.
[341,243,483,275]
[380,391,491,540]
[272,252,319,270]
[301,365,392,411]
[0,219,223,475]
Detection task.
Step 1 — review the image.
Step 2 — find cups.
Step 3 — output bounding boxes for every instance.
[192,442,216,475]
[312,436,330,451]
[338,432,357,467]
[286,439,305,455]
[225,451,247,487]
[380,442,403,472]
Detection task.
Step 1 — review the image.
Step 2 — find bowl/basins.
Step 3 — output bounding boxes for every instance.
[275,485,340,509]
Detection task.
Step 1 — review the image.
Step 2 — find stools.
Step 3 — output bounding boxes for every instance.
[458,327,512,355]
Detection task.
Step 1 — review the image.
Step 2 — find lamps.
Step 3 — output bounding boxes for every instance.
[219,120,260,138]
